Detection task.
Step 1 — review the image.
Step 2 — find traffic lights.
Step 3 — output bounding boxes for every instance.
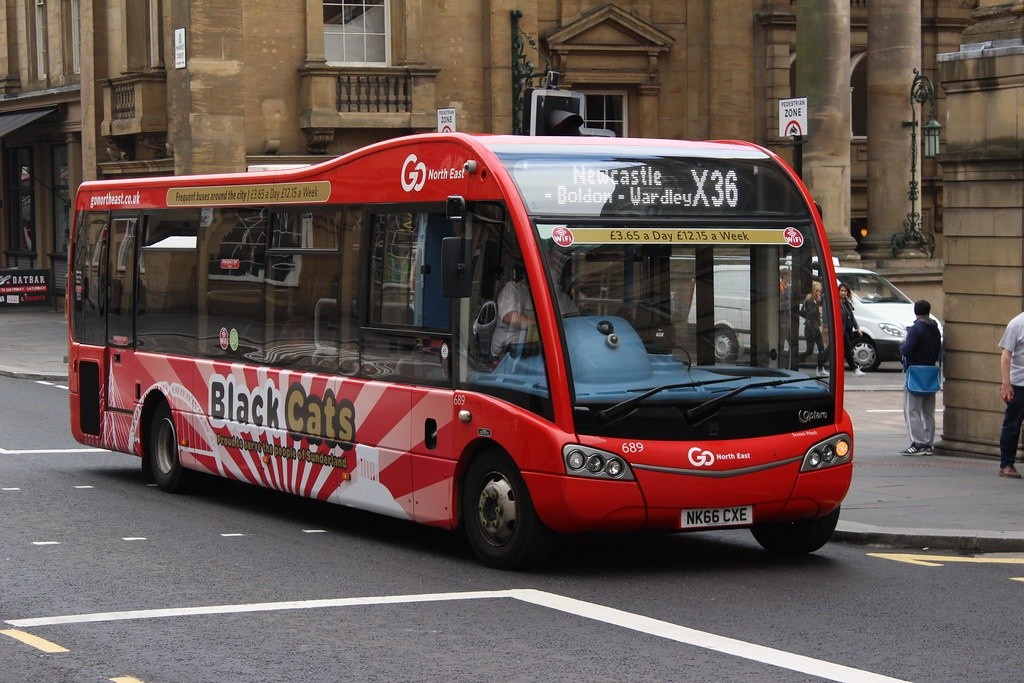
[533,92,585,138]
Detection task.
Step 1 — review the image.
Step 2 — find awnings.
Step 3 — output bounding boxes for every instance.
[0,108,59,137]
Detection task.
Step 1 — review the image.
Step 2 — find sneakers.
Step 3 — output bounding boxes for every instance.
[816,366,829,377]
[900,442,933,455]
[999,465,1022,478]
[854,366,865,377]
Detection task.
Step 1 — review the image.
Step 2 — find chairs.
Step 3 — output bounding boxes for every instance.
[235,316,450,384]
[479,268,511,358]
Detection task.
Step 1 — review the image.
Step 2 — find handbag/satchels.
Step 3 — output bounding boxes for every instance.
[905,365,941,396]
[799,304,806,319]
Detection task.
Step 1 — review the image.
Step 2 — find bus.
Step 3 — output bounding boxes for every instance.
[65,131,855,569]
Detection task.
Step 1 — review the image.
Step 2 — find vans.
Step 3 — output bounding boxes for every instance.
[684,260,943,372]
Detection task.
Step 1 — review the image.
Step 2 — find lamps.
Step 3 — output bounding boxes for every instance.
[887,66,946,260]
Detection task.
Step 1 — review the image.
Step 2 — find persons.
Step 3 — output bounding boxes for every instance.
[779,267,793,369]
[997,311,1024,478]
[824,283,866,376]
[489,264,580,369]
[798,282,829,378]
[899,300,942,456]
